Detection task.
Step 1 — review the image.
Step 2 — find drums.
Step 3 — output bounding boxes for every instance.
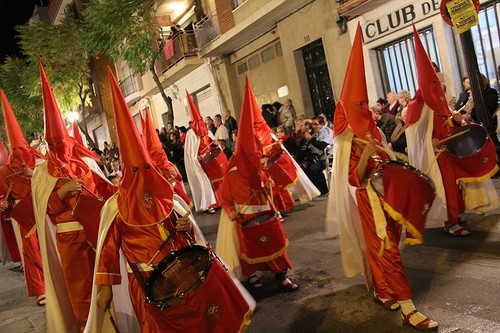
[370,159,434,244]
[141,242,249,333]
[447,122,498,184]
[9,190,37,237]
[237,210,288,265]
[264,150,300,189]
[201,147,230,184]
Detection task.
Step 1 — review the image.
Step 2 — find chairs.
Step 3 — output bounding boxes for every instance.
[322,145,334,191]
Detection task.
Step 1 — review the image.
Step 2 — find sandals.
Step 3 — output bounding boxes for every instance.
[278,278,298,289]
[443,223,469,237]
[400,310,438,330]
[36,294,46,306]
[374,292,400,311]
[247,276,263,287]
[276,214,285,223]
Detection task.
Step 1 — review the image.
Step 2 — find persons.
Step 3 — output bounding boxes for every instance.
[84,151,251,333]
[155,109,238,214]
[262,100,335,223]
[0,141,46,306]
[31,129,115,333]
[327,91,439,333]
[370,91,412,153]
[87,142,122,185]
[218,143,298,291]
[432,61,500,179]
[157,25,188,67]
[404,73,469,237]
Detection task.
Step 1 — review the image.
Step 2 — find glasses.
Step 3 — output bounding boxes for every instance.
[311,122,323,126]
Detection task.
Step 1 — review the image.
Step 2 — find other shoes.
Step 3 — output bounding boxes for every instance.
[20,264,24,272]
[209,209,216,214]
